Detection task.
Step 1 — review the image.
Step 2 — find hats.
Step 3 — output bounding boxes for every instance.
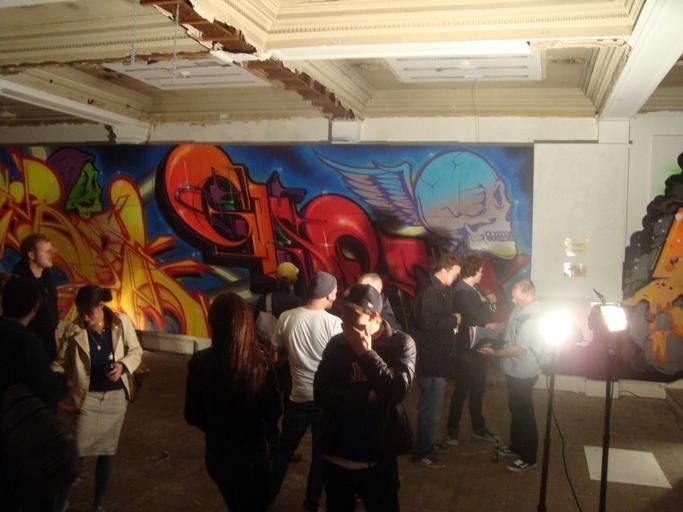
[309,271,337,298]
[344,284,382,314]
[277,262,299,282]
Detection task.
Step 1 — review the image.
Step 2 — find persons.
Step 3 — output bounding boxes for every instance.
[270,270,343,512]
[183,293,283,512]
[1,276,73,511]
[413,253,462,469]
[243,274,278,312]
[357,272,397,330]
[46,284,143,512]
[253,262,306,463]
[312,284,417,512]
[477,278,540,472]
[446,255,501,445]
[9,232,61,359]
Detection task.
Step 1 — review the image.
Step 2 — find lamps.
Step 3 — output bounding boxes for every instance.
[587,288,630,512]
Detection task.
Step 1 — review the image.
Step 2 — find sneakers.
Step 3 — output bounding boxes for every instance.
[418,429,539,472]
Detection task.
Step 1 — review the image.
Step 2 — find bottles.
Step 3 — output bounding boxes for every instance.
[492,439,500,463]
[104,351,114,372]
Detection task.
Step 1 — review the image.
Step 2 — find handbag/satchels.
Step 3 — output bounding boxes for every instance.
[256,312,279,340]
